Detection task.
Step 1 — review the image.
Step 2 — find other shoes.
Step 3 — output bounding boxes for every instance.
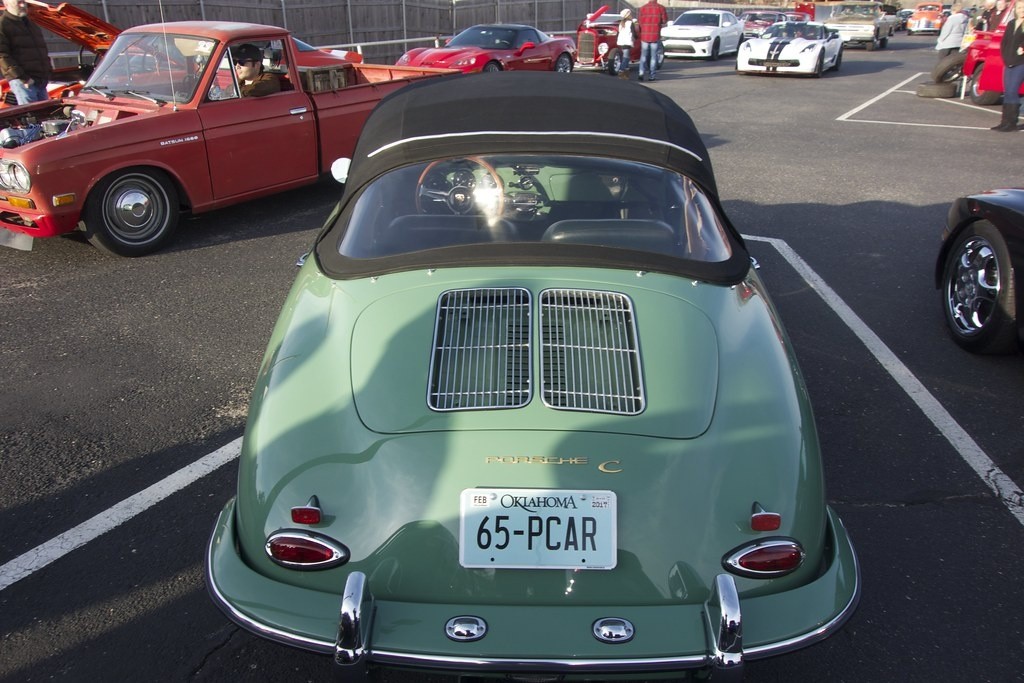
[639,76,643,81]
[648,79,655,81]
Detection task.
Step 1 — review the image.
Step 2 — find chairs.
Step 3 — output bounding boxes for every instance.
[378,213,520,257]
[541,216,683,259]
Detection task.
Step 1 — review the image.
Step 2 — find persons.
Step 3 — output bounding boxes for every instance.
[222,44,280,97]
[0,0,52,105]
[935,0,1010,61]
[616,9,637,80]
[637,0,668,81]
[990,0,1024,131]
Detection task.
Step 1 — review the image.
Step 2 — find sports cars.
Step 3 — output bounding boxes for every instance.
[734,21,844,78]
[392,23,577,75]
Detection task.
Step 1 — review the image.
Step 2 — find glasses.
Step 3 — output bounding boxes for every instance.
[232,59,259,66]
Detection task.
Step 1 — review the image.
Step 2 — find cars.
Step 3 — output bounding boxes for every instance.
[659,9,812,60]
[936,188,1024,360]
[898,2,951,36]
[960,0,1024,104]
[209,73,859,681]
[573,4,665,75]
[0,0,365,110]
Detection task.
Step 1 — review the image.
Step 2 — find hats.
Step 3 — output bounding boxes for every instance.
[620,9,631,18]
[232,44,262,61]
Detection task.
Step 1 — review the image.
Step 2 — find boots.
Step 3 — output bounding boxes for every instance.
[618,72,624,79]
[990,103,1022,131]
[624,69,633,80]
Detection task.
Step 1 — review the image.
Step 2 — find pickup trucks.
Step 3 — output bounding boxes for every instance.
[1,21,465,257]
[823,1,897,48]
